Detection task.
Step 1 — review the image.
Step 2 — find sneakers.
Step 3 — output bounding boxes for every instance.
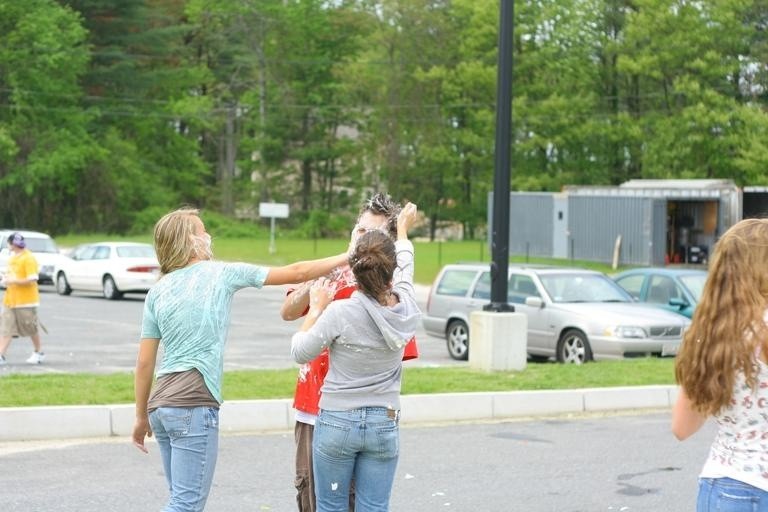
[25,352,46,365]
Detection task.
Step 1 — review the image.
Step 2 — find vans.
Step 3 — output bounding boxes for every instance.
[0,227,65,285]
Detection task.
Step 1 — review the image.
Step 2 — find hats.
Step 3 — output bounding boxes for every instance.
[7,232,26,248]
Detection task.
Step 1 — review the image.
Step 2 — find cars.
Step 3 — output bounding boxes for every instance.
[607,262,710,321]
[52,240,164,302]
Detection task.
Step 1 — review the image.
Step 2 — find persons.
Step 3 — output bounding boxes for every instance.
[281,193,417,511]
[0,232,45,365]
[131,208,355,512]
[669,218,768,512]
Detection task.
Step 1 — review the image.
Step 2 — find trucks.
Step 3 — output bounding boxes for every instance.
[421,255,695,369]
